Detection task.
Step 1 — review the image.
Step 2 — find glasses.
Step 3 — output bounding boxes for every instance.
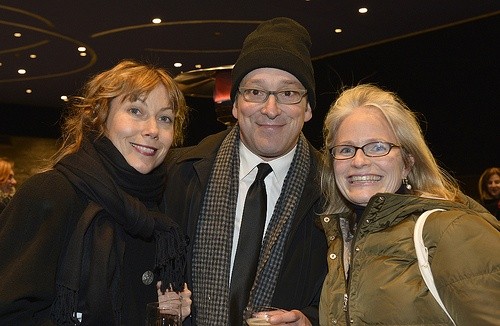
[237,86,307,104]
[329,142,404,159]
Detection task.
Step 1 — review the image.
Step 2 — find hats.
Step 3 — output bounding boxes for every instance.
[229,17,316,110]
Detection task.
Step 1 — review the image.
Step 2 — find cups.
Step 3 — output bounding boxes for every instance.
[245,305,280,326]
[147,302,182,326]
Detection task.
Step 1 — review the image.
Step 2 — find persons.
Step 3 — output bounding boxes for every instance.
[0,159,16,213]
[168,17,327,326]
[477,166,500,220]
[318,84,500,326]
[0,60,193,326]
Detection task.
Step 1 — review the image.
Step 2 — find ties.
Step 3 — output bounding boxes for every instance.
[229,163,275,326]
